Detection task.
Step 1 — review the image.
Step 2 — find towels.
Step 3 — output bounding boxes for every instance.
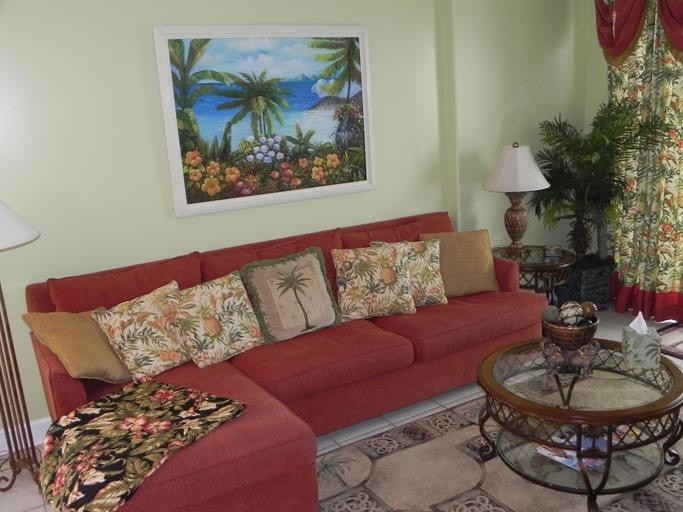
[36,379,247,512]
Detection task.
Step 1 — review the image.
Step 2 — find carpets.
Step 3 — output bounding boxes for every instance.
[655,321,682,360]
[315,366,683,510]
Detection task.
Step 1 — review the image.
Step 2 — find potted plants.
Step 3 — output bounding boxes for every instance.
[532,98,674,314]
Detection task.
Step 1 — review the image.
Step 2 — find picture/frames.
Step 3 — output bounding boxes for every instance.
[153,25,375,218]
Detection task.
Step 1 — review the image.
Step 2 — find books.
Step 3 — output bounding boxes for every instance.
[535,425,620,473]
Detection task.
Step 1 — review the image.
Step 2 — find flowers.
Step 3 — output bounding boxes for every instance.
[182,135,352,199]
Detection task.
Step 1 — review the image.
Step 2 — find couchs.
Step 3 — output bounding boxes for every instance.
[24,210,548,512]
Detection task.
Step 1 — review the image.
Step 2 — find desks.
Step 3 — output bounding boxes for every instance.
[490,242,579,309]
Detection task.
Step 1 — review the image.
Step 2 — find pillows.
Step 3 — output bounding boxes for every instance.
[366,238,450,307]
[240,247,340,344]
[166,267,269,369]
[418,228,500,299]
[331,242,416,327]
[24,305,133,385]
[88,280,195,382]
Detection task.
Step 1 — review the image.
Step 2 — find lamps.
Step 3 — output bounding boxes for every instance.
[0,197,43,511]
[480,143,552,256]
